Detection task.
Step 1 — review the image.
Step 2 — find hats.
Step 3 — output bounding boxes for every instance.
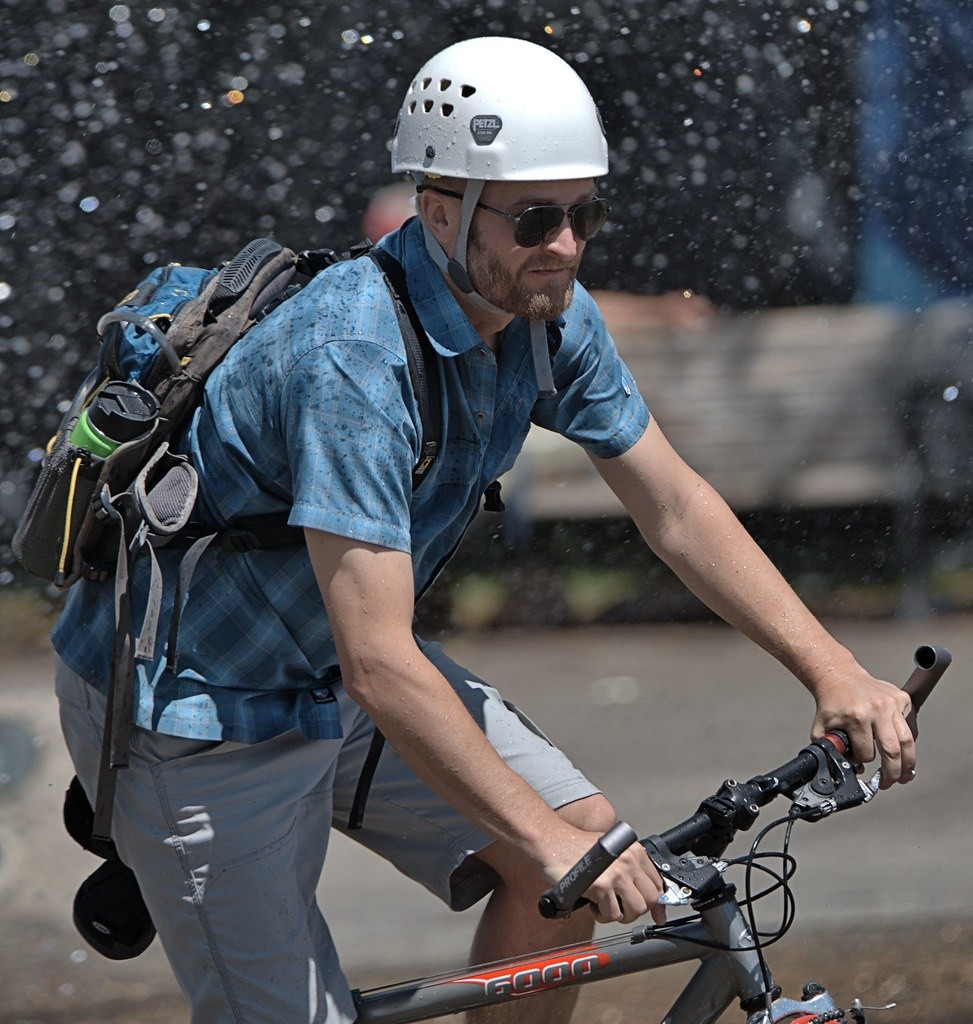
[391,37,609,181]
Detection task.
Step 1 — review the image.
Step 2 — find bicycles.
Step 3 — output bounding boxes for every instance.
[345,644,952,1024]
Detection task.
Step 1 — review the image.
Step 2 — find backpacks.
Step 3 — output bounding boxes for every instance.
[13,238,566,588]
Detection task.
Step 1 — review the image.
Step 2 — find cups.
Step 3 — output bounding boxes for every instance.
[70,381,163,458]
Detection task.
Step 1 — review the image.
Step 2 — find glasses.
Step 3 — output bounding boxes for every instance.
[417,184,612,249]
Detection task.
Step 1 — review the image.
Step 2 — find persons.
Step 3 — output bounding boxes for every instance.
[52,37,917,1024]
